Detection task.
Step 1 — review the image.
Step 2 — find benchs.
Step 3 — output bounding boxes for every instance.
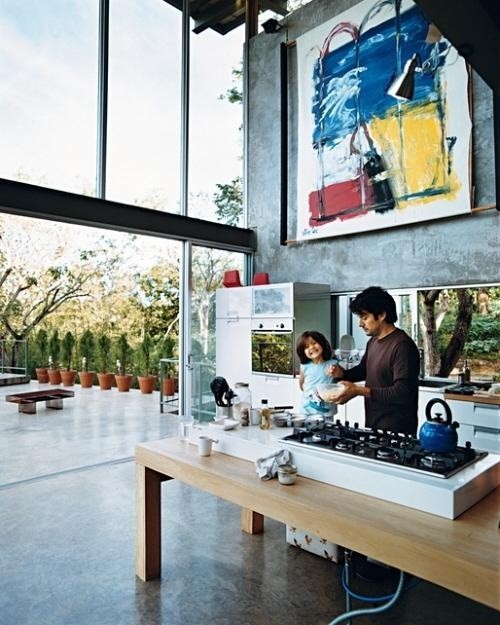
[5,388,74,414]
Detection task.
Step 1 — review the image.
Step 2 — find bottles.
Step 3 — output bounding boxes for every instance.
[233,382,252,427]
[260,399,271,430]
[458,358,471,386]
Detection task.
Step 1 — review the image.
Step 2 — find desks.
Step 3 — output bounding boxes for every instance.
[132,436,500,625]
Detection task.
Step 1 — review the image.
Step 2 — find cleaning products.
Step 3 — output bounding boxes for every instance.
[462,360,470,385]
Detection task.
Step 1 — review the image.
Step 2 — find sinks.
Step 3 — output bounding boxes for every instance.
[417,378,458,388]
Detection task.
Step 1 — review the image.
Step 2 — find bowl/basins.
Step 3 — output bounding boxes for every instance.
[270,412,325,428]
[315,383,347,405]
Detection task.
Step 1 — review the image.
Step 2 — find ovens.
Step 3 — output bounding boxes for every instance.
[250,317,298,377]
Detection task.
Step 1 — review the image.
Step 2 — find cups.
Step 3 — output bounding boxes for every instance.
[197,435,214,457]
[214,404,233,422]
[277,464,298,486]
[248,408,261,426]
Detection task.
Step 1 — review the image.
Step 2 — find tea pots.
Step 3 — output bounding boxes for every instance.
[418,398,460,452]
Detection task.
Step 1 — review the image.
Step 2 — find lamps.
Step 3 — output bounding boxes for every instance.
[260,18,284,34]
[385,40,461,101]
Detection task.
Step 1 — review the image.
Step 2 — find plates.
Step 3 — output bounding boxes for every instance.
[209,418,240,430]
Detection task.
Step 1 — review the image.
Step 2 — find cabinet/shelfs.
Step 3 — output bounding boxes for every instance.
[214,281,500,455]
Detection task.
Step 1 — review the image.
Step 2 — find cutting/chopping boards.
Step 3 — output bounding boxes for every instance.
[444,391,500,406]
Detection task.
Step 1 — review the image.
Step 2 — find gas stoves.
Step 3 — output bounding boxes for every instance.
[414,379,457,393]
[279,422,489,479]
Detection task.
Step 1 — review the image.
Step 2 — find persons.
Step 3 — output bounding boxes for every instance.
[326,285,420,586]
[295,331,337,424]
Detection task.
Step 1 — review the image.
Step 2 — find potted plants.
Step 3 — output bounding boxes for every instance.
[35,330,177,395]
